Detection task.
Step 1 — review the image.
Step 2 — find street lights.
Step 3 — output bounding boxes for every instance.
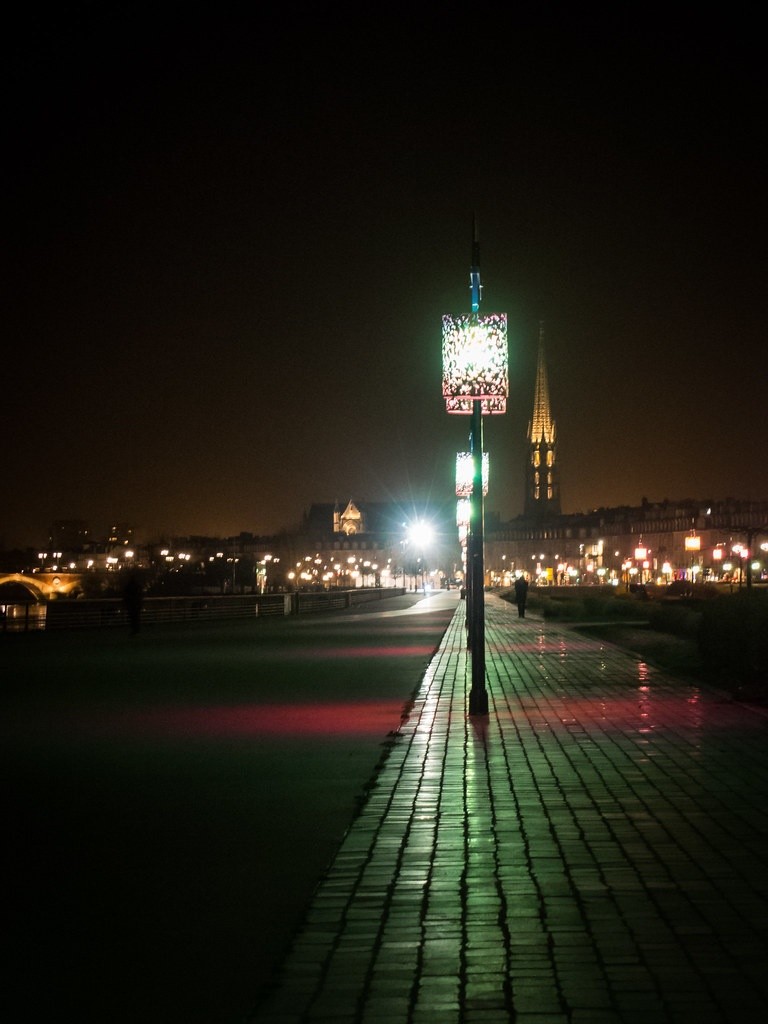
[661,563,670,587]
[732,543,748,590]
[53,552,62,572]
[39,552,47,572]
[440,310,511,712]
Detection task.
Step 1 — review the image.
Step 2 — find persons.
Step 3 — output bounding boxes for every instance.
[514,576,529,618]
